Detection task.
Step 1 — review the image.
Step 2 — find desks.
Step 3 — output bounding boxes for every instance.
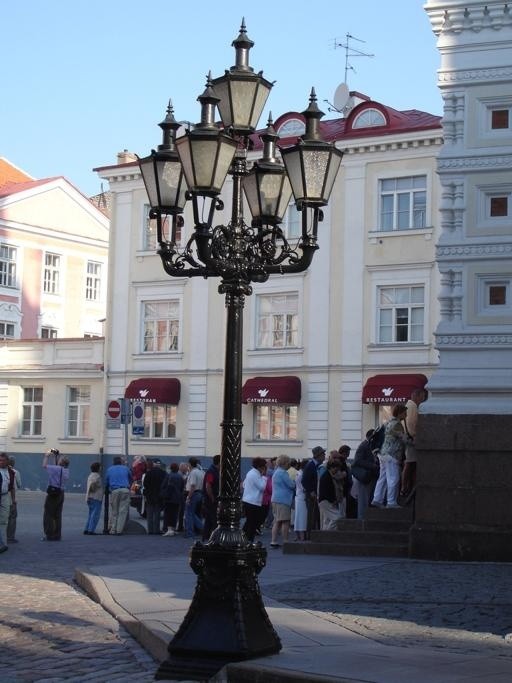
[126,15,348,657]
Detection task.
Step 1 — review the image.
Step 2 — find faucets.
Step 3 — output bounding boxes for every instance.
[399,490,408,496]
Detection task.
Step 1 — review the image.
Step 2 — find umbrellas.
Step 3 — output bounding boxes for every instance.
[162,526,175,537]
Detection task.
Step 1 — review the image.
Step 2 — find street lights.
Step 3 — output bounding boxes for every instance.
[350,460,377,485]
[47,485,61,496]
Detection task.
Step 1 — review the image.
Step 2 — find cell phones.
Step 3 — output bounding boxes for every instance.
[51,448,60,455]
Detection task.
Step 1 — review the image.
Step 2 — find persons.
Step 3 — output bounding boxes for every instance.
[241,389,425,548]
[86,455,220,535]
[0,452,13,554]
[40,448,70,542]
[7,455,22,543]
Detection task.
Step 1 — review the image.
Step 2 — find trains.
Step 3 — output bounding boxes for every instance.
[107,399,120,419]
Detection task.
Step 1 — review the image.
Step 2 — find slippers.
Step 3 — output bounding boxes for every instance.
[367,423,385,456]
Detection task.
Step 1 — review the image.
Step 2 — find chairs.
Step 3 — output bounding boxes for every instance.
[269,542,282,549]
[255,527,264,536]
[84,529,96,535]
[370,500,386,510]
[386,503,404,509]
[6,537,19,543]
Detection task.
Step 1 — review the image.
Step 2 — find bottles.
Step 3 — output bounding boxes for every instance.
[312,445,326,459]
[153,459,161,466]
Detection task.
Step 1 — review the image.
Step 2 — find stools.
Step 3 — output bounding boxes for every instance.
[1,492,8,495]
[113,486,130,489]
